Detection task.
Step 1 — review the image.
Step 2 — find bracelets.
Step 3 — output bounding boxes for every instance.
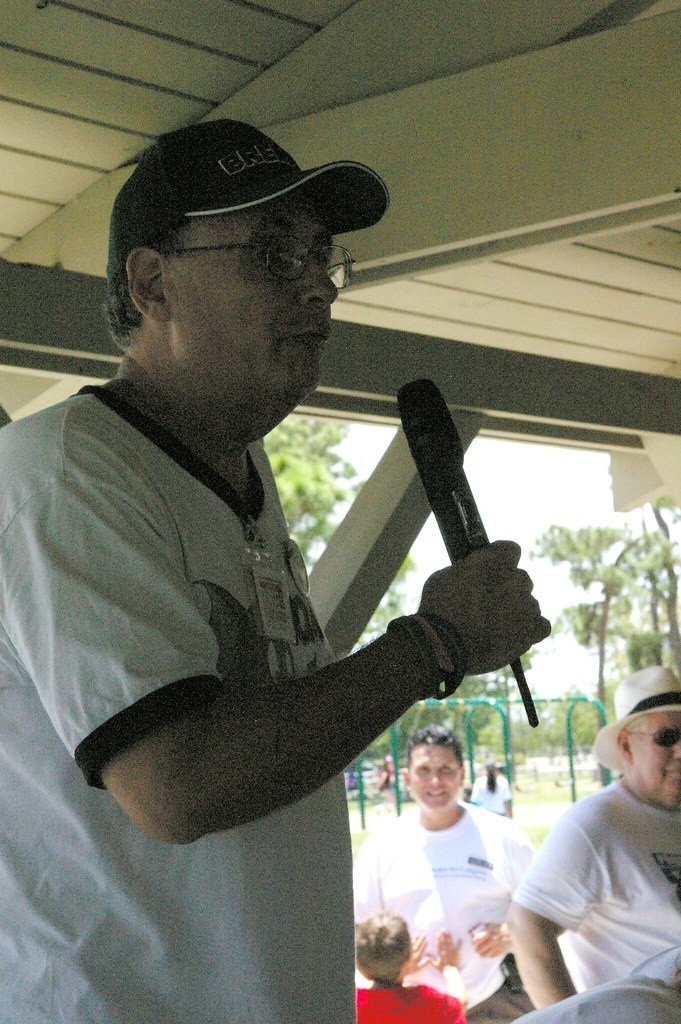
[388,614,466,701]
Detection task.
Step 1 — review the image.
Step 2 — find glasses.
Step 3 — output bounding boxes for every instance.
[626,725,681,748]
[161,232,355,289]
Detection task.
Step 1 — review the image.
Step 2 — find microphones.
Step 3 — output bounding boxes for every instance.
[398,377,539,728]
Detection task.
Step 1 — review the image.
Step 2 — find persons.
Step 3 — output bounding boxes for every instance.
[0,118,551,1024]
[348,725,681,1024]
[507,666,680,1010]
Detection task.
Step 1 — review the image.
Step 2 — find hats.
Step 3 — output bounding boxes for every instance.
[594,667,681,771]
[106,116,391,284]
